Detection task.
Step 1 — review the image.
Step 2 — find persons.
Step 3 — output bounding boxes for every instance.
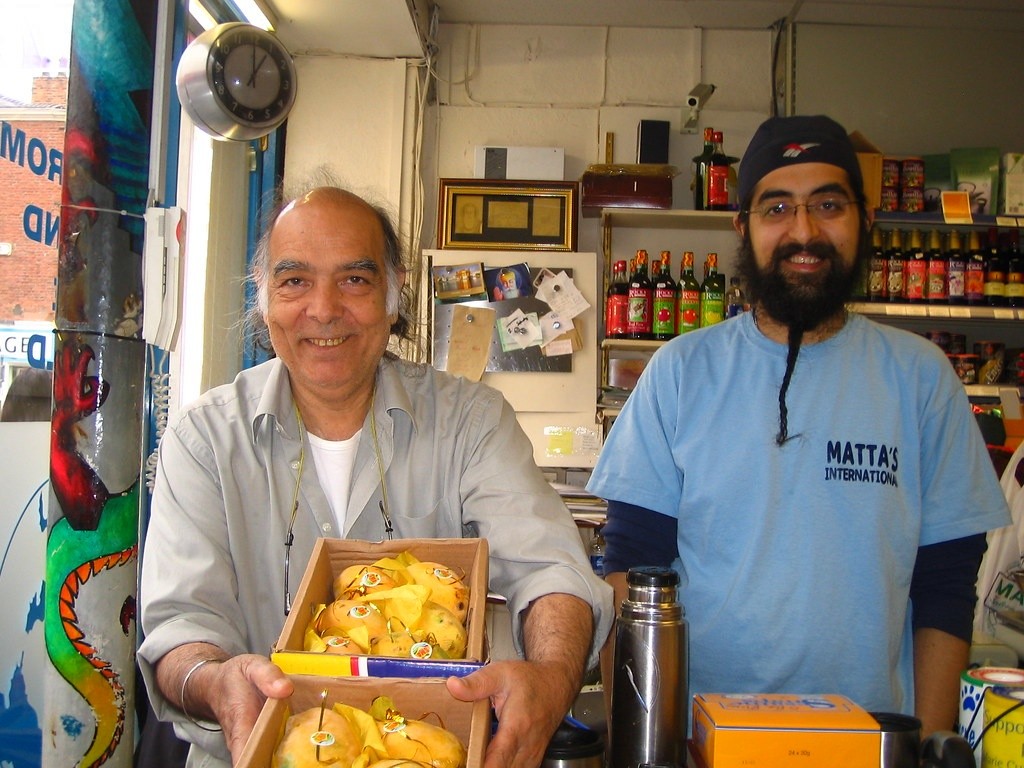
[131,188,616,768]
[579,116,1013,768]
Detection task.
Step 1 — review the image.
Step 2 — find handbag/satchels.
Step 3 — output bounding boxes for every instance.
[972,441,1024,656]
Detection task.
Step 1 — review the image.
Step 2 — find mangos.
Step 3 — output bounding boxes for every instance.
[272,707,467,768]
[315,562,470,658]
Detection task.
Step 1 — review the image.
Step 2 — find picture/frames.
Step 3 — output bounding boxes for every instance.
[436,177,579,253]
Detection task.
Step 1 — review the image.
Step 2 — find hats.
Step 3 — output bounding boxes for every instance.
[737,115,863,212]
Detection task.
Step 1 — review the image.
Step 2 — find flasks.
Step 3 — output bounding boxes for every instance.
[604,565,691,768]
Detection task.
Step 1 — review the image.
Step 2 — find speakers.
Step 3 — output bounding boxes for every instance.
[637,120,669,166]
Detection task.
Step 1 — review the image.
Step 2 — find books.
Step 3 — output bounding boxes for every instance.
[548,481,608,525]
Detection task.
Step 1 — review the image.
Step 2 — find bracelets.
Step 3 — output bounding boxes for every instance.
[181,657,224,732]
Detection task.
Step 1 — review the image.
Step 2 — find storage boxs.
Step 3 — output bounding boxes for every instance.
[692,692,885,768]
[848,128,883,207]
[580,173,673,217]
[230,536,492,767]
[1000,153,1024,217]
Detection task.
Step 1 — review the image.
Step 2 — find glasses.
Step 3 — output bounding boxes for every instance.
[742,199,859,220]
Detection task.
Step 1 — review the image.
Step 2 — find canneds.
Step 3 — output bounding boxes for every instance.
[909,330,1024,385]
[878,157,925,213]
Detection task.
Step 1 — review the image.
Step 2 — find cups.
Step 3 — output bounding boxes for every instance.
[866,711,923,768]
[540,724,604,768]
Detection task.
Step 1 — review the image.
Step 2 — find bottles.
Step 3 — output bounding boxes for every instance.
[880,153,925,212]
[924,330,1024,388]
[692,127,714,211]
[866,226,1024,310]
[606,249,745,342]
[705,131,729,211]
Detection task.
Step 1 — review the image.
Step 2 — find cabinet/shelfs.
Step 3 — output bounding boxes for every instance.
[597,206,1024,419]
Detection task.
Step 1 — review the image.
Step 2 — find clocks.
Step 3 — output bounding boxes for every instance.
[175,23,300,143]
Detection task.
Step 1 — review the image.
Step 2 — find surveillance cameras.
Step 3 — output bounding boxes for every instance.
[687,83,713,109]
